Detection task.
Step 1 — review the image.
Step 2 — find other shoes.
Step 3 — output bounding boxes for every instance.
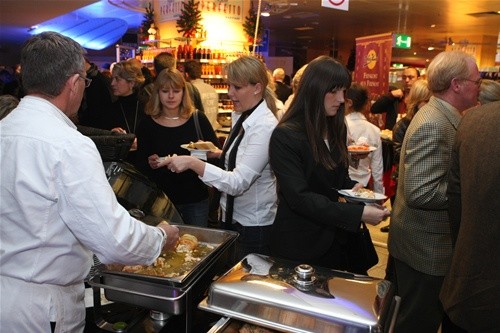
[380,225,390,232]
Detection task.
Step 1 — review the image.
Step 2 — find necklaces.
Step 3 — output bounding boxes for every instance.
[164,116,179,120]
[118,100,136,135]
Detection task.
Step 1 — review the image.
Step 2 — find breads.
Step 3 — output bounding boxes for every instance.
[179,234,197,249]
[194,141,215,150]
[380,128,392,140]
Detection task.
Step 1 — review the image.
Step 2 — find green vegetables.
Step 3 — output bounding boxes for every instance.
[137,244,214,276]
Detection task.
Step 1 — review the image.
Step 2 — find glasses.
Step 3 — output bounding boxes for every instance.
[70,71,93,88]
[458,77,483,87]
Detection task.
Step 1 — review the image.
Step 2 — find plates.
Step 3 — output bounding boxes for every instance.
[338,189,388,205]
[180,144,219,151]
[347,146,376,154]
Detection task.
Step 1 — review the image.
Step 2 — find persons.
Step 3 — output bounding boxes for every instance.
[269,56,390,275]
[0,32,178,333]
[0,51,500,195]
[158,56,281,252]
[381,50,482,333]
[136,68,220,227]
[440,100,500,333]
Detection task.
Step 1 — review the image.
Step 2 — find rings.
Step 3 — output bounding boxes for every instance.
[171,168,175,173]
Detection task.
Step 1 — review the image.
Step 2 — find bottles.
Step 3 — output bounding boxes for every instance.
[136,23,265,110]
[388,71,398,84]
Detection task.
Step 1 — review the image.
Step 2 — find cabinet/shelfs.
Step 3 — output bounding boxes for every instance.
[139,45,264,133]
[348,65,427,200]
